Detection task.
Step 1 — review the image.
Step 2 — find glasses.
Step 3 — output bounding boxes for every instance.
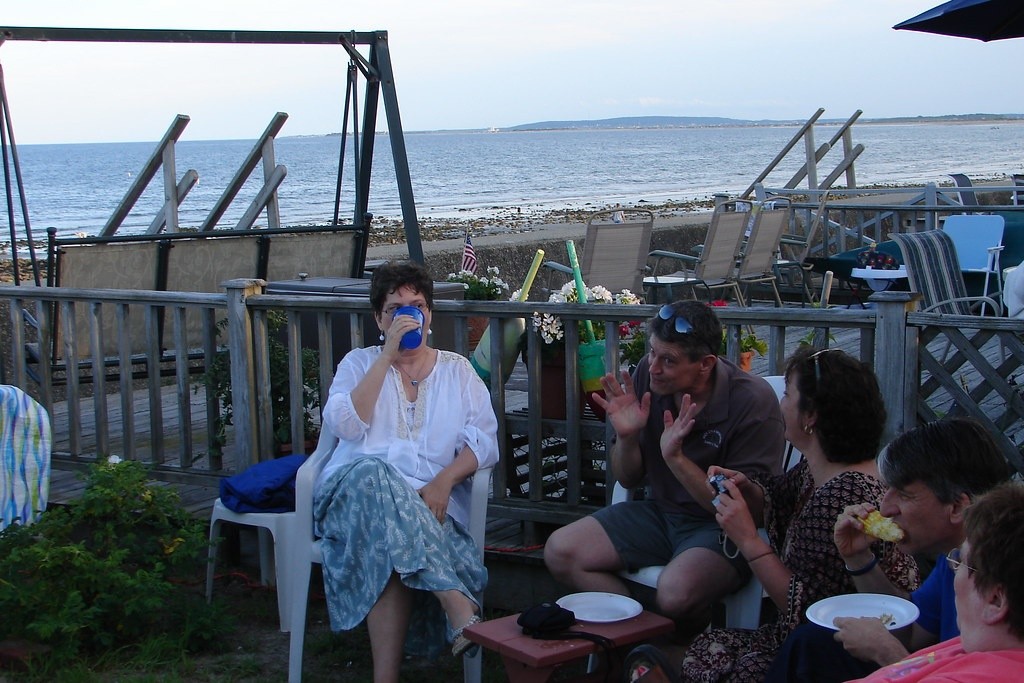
[656,303,701,340]
[381,303,429,314]
[811,348,844,392]
[946,548,979,573]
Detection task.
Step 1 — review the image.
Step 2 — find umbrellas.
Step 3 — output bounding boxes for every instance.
[892,0,1024,42]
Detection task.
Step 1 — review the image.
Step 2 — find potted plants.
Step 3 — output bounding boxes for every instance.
[190,308,320,463]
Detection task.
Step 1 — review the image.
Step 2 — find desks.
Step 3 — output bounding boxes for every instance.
[463,607,674,683]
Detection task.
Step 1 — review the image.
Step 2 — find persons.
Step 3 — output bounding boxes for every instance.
[544,300,787,646]
[313,257,499,683]
[683,343,920,683]
[622,481,1024,683]
[611,203,626,223]
[763,417,1013,683]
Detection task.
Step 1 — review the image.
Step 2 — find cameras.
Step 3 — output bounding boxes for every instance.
[707,472,733,508]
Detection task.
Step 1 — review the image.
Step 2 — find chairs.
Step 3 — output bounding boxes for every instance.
[287,417,495,683]
[899,215,1005,318]
[733,190,829,307]
[886,230,1007,366]
[1005,172,1024,206]
[586,373,787,673]
[690,197,792,311]
[942,173,981,216]
[644,199,755,337]
[541,208,654,300]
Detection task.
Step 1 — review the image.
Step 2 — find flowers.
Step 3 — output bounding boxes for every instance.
[445,266,509,301]
[619,300,768,377]
[531,279,642,345]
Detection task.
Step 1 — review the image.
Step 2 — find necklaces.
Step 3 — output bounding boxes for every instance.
[394,349,429,386]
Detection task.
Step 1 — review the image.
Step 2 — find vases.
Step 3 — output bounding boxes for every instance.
[577,339,605,393]
[739,349,753,372]
[467,315,489,351]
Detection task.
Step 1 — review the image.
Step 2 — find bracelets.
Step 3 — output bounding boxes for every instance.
[845,553,876,576]
[747,551,777,564]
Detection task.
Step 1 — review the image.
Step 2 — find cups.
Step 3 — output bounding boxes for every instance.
[392,306,425,349]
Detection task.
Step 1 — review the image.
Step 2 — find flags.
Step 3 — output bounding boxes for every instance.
[462,228,478,274]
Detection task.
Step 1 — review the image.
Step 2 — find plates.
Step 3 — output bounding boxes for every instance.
[806,593,920,631]
[556,591,643,622]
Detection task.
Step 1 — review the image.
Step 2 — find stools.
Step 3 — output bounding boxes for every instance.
[206,497,298,632]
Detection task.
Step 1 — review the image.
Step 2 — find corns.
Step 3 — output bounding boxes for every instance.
[856,507,904,543]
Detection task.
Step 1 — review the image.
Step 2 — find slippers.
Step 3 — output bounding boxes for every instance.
[450,613,482,654]
